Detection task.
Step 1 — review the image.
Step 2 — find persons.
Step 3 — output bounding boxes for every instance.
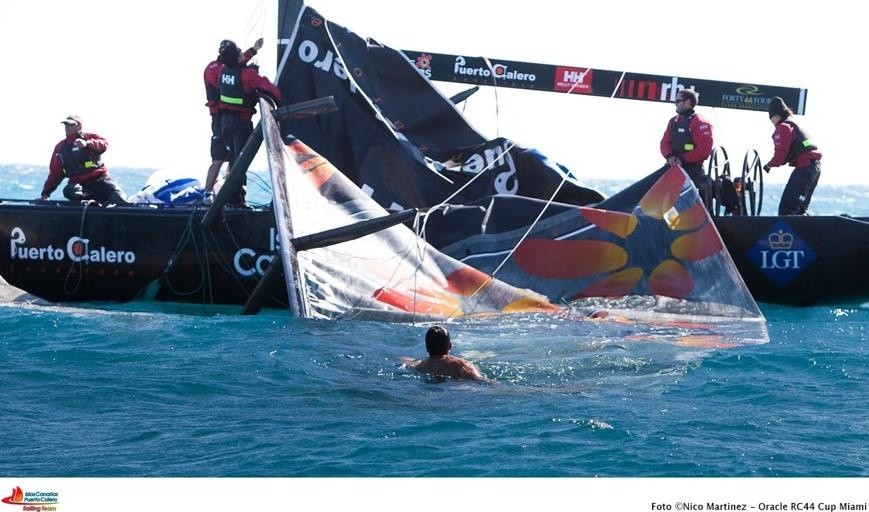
[205,44,283,207]
[200,37,266,206]
[29,114,135,207]
[659,90,712,215]
[762,96,824,215]
[399,323,489,381]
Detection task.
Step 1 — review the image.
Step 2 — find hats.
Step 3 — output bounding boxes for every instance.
[768,96,787,115]
[61,114,83,128]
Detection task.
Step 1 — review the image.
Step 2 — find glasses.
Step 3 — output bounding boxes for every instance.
[675,96,690,104]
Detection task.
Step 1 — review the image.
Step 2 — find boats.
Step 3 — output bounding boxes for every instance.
[0,194,869,315]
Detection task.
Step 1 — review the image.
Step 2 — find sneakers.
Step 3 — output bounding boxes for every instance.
[201,191,215,205]
